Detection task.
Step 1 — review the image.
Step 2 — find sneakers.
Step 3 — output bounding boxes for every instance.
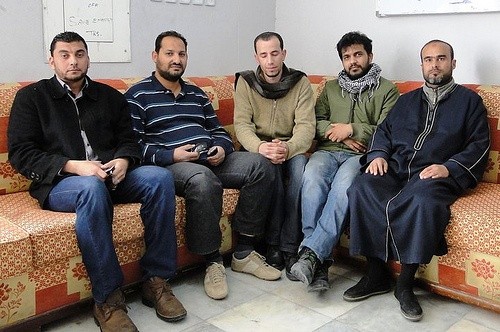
[231,250,283,280]
[205,261,229,299]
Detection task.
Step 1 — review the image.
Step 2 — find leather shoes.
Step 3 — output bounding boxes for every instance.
[266,246,284,271]
[285,252,300,281]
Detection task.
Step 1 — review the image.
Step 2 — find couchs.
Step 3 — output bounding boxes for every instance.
[0,74,242,332]
[306,74,500,312]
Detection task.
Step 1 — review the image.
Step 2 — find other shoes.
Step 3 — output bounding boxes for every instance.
[290,249,321,286]
[141,276,187,321]
[343,274,391,300]
[307,262,329,293]
[394,283,425,320]
[92,287,139,332]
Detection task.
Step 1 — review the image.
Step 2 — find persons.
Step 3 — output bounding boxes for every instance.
[232,31,316,281]
[341,39,492,320]
[121,30,283,300]
[289,31,400,293]
[7,31,188,332]
